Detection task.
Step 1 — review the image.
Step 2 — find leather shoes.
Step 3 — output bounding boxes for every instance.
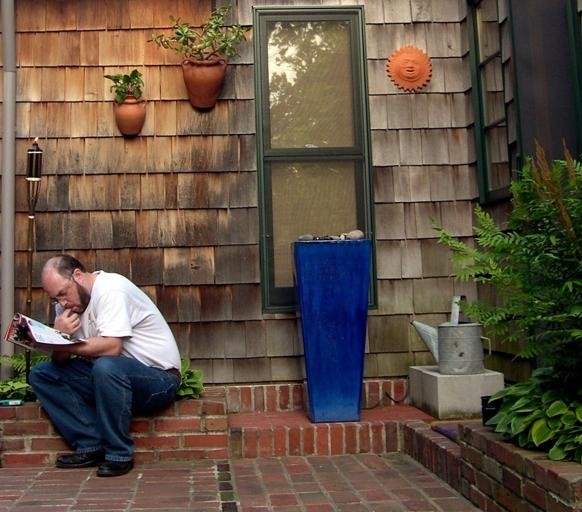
[95,460,134,478]
[54,452,103,469]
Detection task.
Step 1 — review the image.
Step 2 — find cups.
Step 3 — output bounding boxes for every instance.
[480,395,504,426]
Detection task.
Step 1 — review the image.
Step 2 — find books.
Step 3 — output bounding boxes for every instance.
[4,313,87,355]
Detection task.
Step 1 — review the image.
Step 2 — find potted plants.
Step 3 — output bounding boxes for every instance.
[147,3,247,108]
[104,70,148,135]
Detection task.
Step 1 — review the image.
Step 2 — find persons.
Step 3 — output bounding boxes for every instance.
[30,255,181,475]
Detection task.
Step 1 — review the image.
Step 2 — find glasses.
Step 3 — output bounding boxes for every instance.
[51,273,74,304]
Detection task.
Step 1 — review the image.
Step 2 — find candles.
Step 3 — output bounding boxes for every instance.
[26,135,43,182]
